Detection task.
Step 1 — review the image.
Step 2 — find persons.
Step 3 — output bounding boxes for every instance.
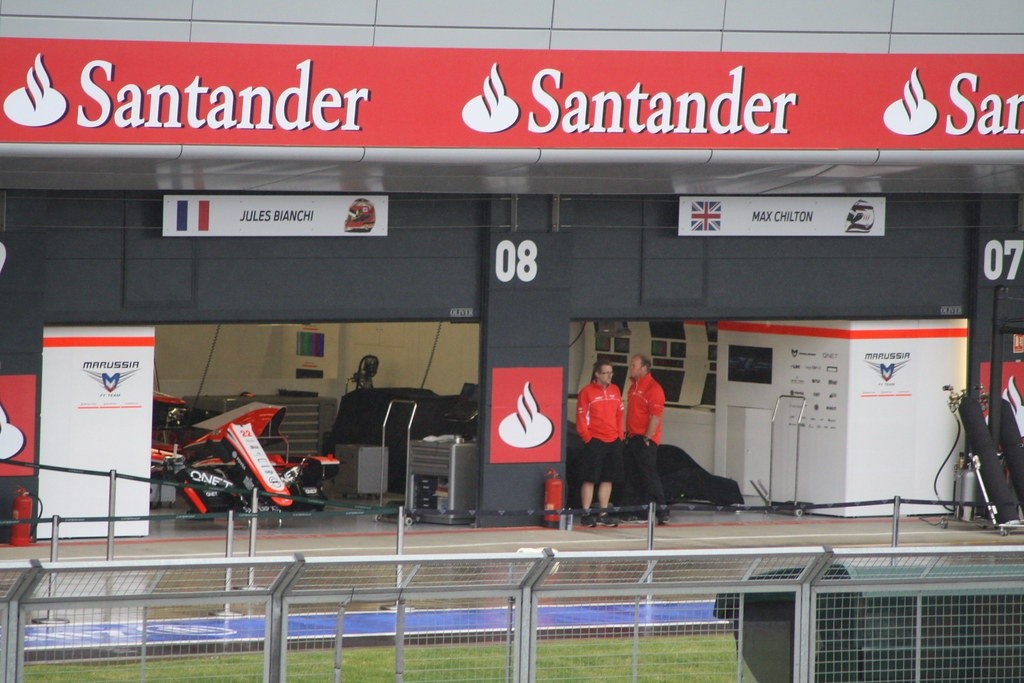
[628,354,669,523]
[577,361,625,527]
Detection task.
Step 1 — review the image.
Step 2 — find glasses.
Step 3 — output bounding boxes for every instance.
[600,370,613,377]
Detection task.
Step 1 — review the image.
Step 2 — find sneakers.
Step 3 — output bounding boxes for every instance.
[658,510,671,523]
[621,510,649,524]
[596,514,618,526]
[580,514,596,526]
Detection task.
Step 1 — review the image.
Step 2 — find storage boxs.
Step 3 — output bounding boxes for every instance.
[414,476,449,509]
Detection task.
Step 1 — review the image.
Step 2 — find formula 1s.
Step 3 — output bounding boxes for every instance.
[150,387,341,520]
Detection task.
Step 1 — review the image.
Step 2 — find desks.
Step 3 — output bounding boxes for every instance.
[406,441,476,525]
